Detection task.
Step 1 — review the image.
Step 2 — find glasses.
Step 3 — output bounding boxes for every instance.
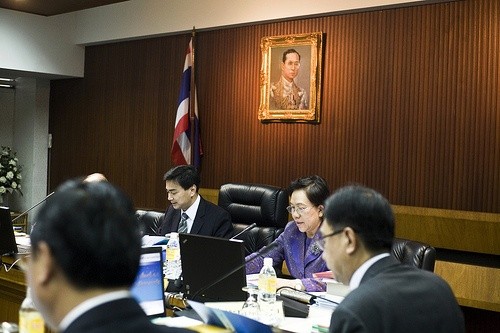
[314,228,356,252]
[286,204,315,214]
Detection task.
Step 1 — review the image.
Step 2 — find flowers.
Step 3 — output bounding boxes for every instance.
[0,145,27,203]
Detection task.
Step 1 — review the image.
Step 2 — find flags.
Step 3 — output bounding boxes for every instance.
[171,36,201,174]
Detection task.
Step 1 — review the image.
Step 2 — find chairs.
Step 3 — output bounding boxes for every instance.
[219,181,287,280]
[134,209,167,237]
[391,239,434,270]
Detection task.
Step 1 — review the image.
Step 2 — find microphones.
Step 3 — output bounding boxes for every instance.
[231,223,256,239]
[11,192,56,222]
[191,241,278,301]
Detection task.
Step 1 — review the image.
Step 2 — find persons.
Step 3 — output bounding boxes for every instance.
[27,180,199,333]
[83,172,107,183]
[160,165,234,240]
[246,175,331,291]
[319,185,464,333]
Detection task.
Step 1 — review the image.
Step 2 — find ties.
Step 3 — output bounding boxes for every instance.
[178,213,190,233]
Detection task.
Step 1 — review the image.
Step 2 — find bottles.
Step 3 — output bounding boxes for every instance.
[256,257,278,314]
[164,232,181,279]
[18,287,45,333]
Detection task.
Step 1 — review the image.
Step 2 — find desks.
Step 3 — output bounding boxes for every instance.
[0,237,344,333]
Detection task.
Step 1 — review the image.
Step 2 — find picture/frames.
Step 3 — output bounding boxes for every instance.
[258,31,324,123]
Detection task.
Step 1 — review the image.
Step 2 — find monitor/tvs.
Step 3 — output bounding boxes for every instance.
[130,247,167,321]
[179,232,250,304]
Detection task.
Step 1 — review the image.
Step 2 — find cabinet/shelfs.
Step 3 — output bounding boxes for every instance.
[11,212,27,233]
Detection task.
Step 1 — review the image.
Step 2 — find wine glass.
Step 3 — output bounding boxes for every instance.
[239,285,260,321]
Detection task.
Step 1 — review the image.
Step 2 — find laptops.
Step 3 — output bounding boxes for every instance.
[0,207,31,256]
